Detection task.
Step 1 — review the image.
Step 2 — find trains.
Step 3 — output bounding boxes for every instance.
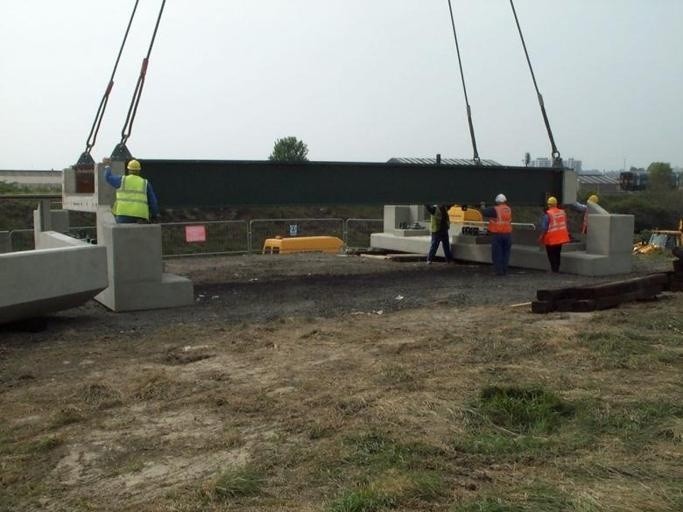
[619,171,680,192]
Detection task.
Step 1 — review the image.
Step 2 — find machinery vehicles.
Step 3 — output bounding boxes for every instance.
[633,220,683,258]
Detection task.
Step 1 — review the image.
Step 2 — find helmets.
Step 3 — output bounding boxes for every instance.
[495,194,507,203]
[126,159,141,171]
[586,195,598,203]
[547,196,558,206]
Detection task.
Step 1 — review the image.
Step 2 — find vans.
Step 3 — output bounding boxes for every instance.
[262,235,345,255]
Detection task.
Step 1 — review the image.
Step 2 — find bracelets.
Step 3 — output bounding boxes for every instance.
[480,203,485,205]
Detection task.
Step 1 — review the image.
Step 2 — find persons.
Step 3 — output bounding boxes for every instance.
[104,159,161,222]
[480,194,514,275]
[581,194,600,234]
[664,234,677,248]
[423,200,458,265]
[537,196,571,273]
[649,227,665,247]
